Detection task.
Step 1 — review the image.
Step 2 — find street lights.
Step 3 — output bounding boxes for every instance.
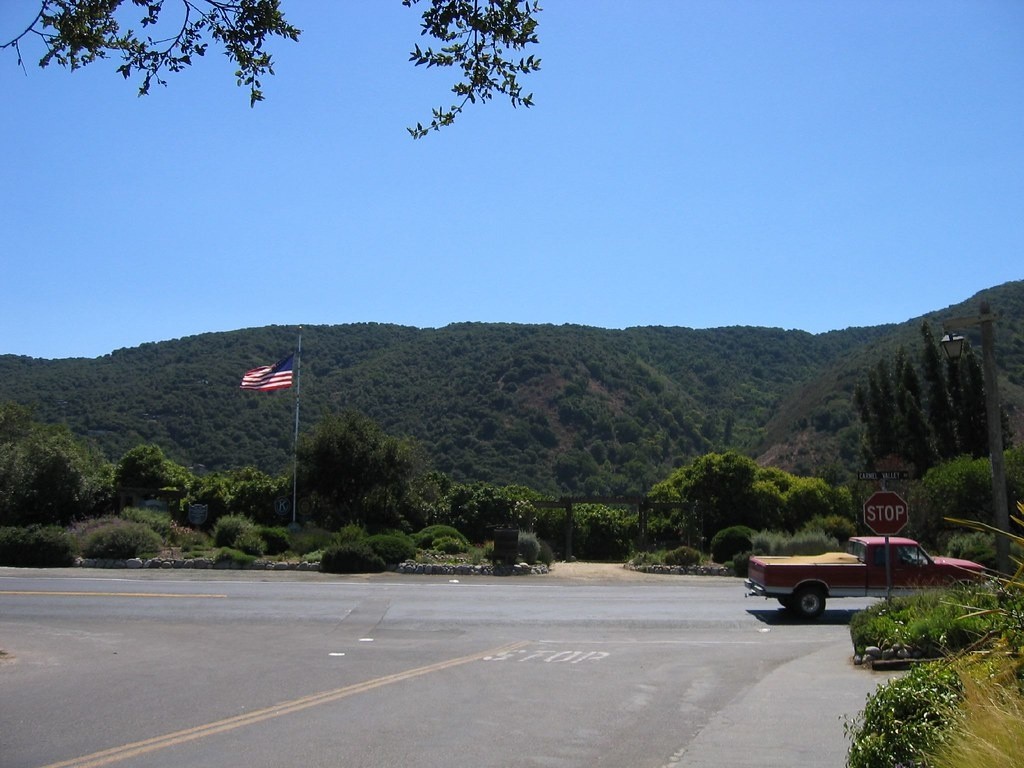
[941,297,1013,578]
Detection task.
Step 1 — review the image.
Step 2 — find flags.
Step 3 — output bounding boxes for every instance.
[239,352,294,391]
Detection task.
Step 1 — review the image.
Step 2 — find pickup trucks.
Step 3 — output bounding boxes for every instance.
[746,534,989,618]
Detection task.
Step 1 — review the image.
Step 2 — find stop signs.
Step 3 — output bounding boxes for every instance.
[862,490,910,535]
